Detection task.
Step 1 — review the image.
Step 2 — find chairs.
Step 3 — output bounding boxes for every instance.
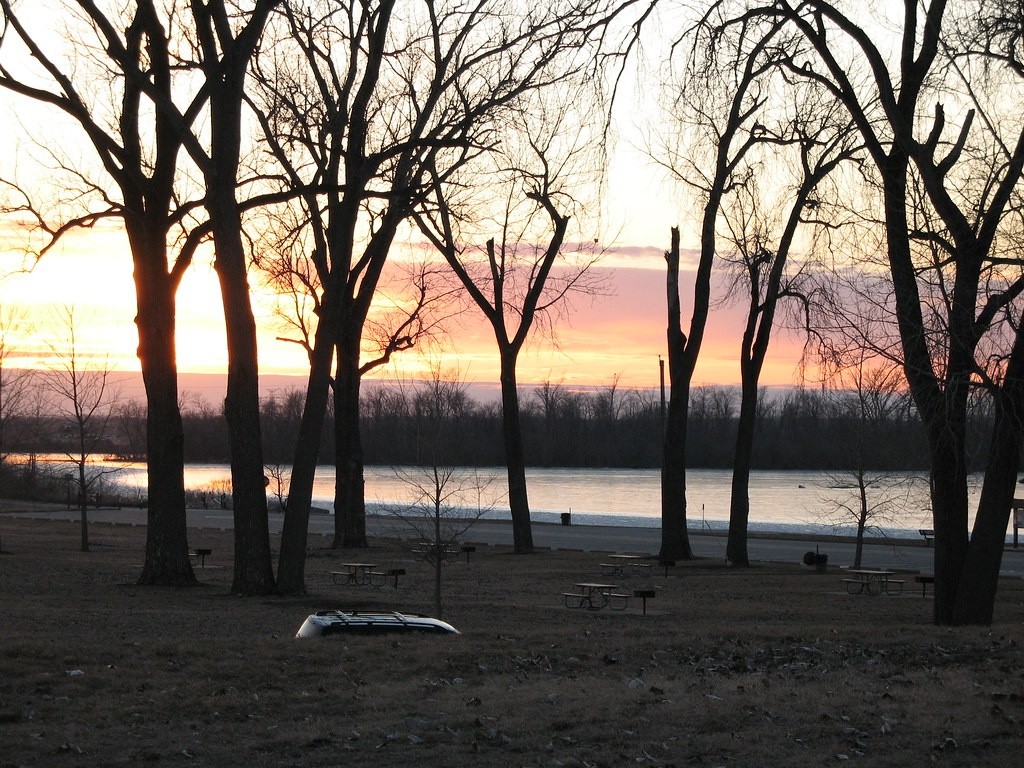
[919,530,935,547]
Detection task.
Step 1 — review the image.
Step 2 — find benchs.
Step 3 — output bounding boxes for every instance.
[332,550,459,589]
[189,547,212,569]
[561,563,652,610]
[843,578,907,596]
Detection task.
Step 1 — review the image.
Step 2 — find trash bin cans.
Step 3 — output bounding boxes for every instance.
[561,512,570,525]
[815,554,829,574]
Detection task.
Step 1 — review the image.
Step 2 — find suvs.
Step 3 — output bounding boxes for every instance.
[295,609,462,640]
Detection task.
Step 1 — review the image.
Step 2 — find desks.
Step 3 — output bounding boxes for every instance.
[846,569,896,595]
[342,563,376,586]
[608,555,643,576]
[420,543,452,546]
[575,583,619,609]
